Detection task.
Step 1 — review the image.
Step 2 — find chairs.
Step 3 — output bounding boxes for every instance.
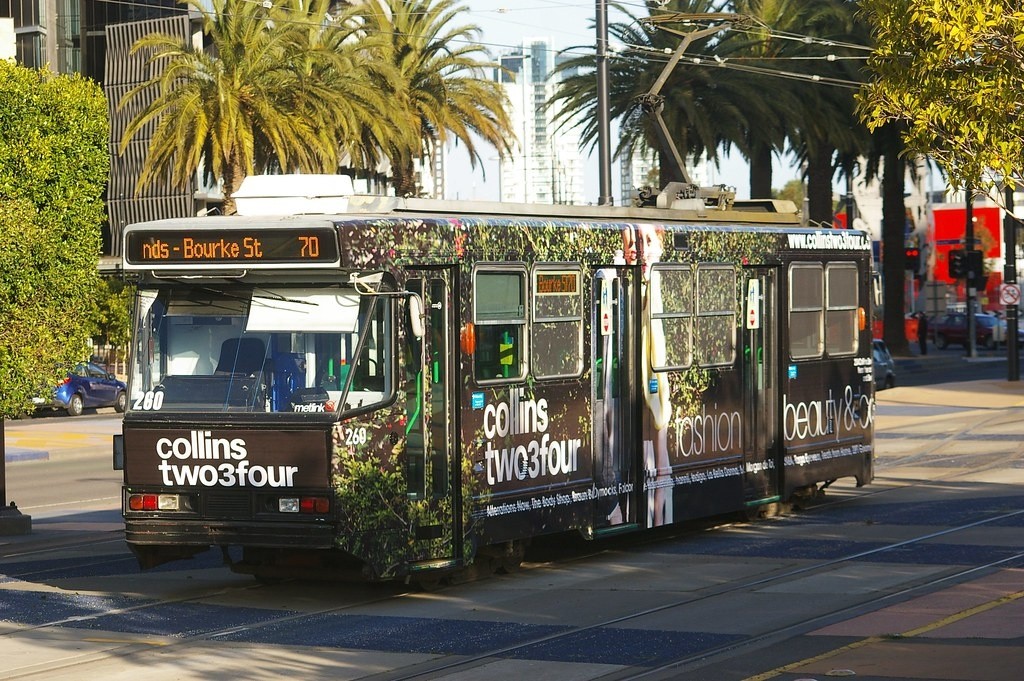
[211,338,267,409]
[320,363,355,393]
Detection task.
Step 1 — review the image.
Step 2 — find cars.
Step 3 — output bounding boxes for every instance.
[25,360,127,416]
[872,338,896,392]
[924,309,1024,348]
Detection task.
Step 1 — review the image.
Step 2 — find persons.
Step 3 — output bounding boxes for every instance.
[917,310,928,355]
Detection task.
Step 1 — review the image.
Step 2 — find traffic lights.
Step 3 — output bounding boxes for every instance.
[948,249,958,278]
[905,248,919,269]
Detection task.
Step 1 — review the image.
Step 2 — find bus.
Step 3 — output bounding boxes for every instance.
[93,173,877,593]
